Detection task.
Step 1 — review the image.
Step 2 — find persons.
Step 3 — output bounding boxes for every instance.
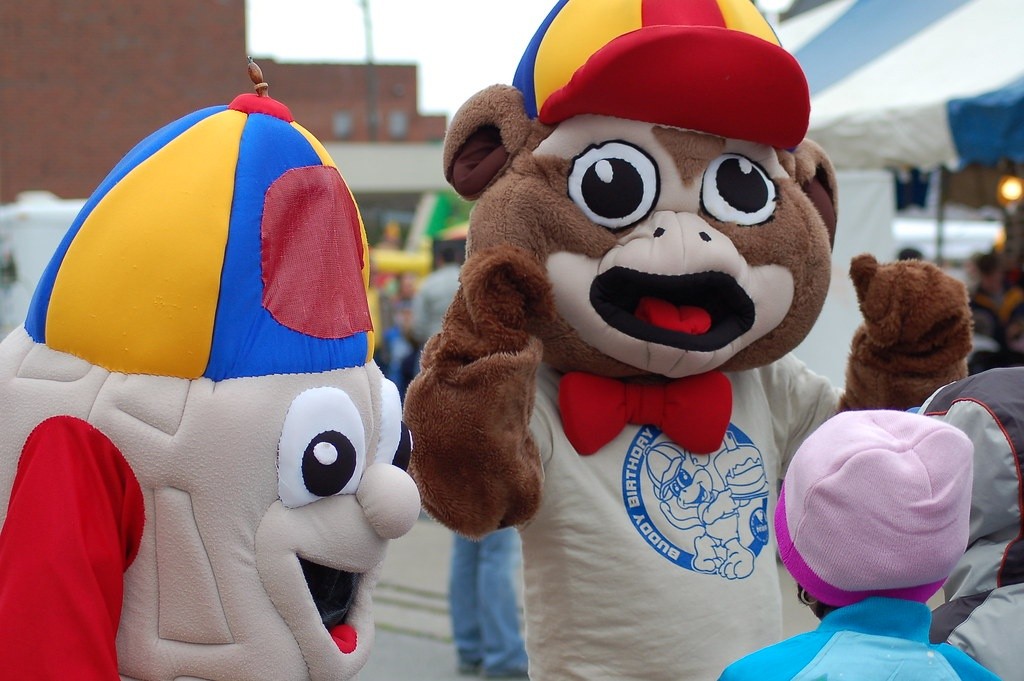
[914,359,1023,678]
[715,408,1003,680]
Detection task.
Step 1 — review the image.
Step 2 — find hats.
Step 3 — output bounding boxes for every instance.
[780,409,972,608]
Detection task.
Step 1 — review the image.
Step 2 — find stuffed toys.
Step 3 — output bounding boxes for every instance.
[0,54,420,677]
[404,0,979,679]
